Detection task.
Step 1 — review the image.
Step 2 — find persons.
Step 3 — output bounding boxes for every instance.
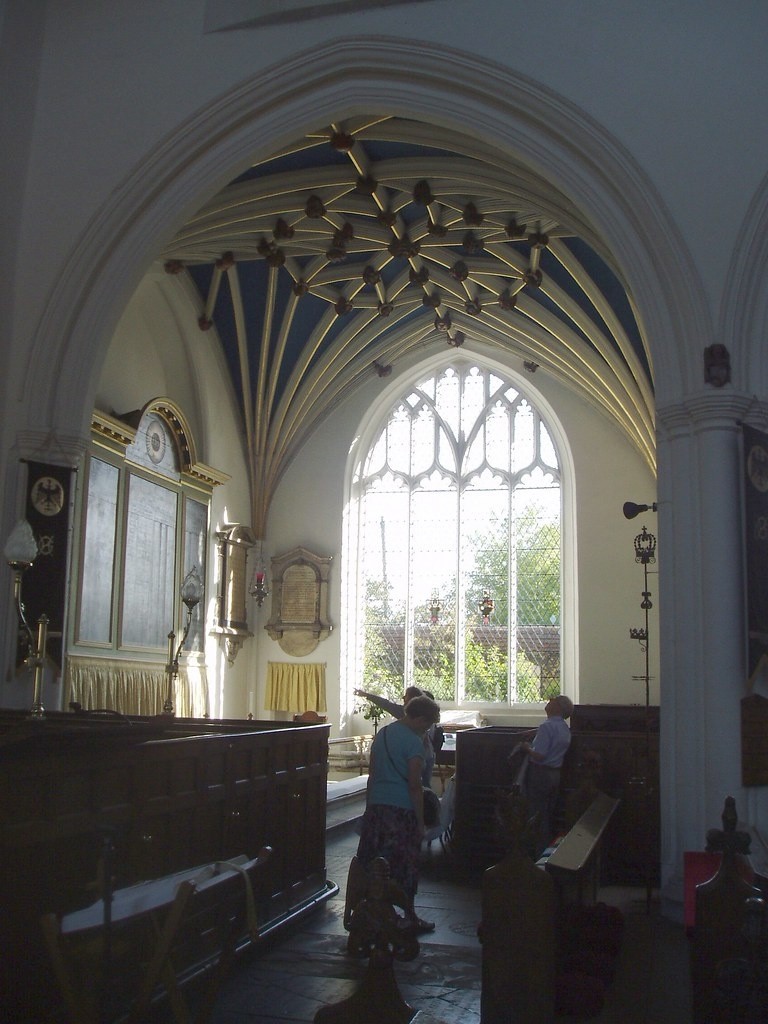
[519,696,575,863]
[356,686,436,790]
[356,695,440,934]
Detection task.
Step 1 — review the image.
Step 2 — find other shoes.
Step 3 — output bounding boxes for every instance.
[405,916,435,931]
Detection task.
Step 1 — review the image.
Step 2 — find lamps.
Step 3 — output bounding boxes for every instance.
[2,521,49,721]
[248,541,273,608]
[164,565,205,717]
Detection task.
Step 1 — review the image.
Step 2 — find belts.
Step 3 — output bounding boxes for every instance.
[528,762,553,770]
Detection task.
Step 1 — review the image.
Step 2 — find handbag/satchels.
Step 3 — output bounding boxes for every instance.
[422,785,441,829]
